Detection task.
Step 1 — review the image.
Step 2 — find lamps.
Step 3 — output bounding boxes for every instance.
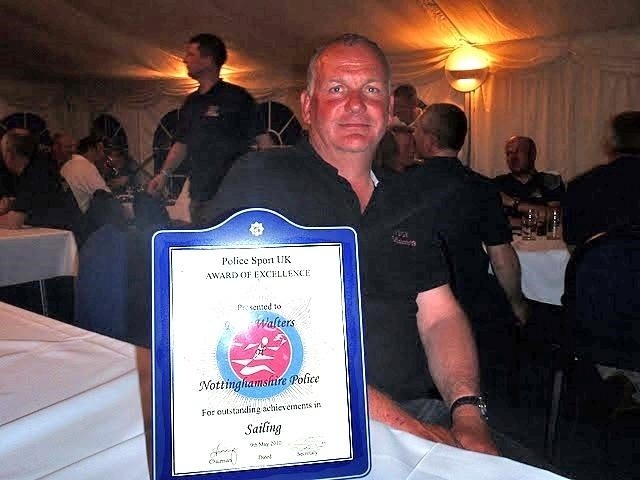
[443,44,491,92]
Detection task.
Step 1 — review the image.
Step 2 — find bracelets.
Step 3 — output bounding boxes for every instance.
[512,194,521,209]
[158,167,169,178]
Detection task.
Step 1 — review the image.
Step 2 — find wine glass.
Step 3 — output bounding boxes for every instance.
[104,156,120,177]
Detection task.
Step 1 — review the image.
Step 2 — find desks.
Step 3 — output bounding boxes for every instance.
[0,300,576,478]
[0,228,82,317]
[478,229,571,304]
[115,191,175,221]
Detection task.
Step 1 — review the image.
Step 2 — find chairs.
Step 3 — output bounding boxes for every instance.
[547,226,640,477]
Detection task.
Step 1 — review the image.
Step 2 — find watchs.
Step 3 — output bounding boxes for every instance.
[444,391,495,422]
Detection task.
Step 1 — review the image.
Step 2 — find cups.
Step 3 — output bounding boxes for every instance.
[546,206,562,240]
[521,209,536,240]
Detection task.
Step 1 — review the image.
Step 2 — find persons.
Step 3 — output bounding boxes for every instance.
[374,124,423,182]
[102,146,144,188]
[492,135,564,230]
[60,136,111,213]
[206,32,499,455]
[396,105,552,407]
[561,110,640,424]
[1,129,91,323]
[389,85,425,130]
[48,131,79,167]
[145,32,257,223]
